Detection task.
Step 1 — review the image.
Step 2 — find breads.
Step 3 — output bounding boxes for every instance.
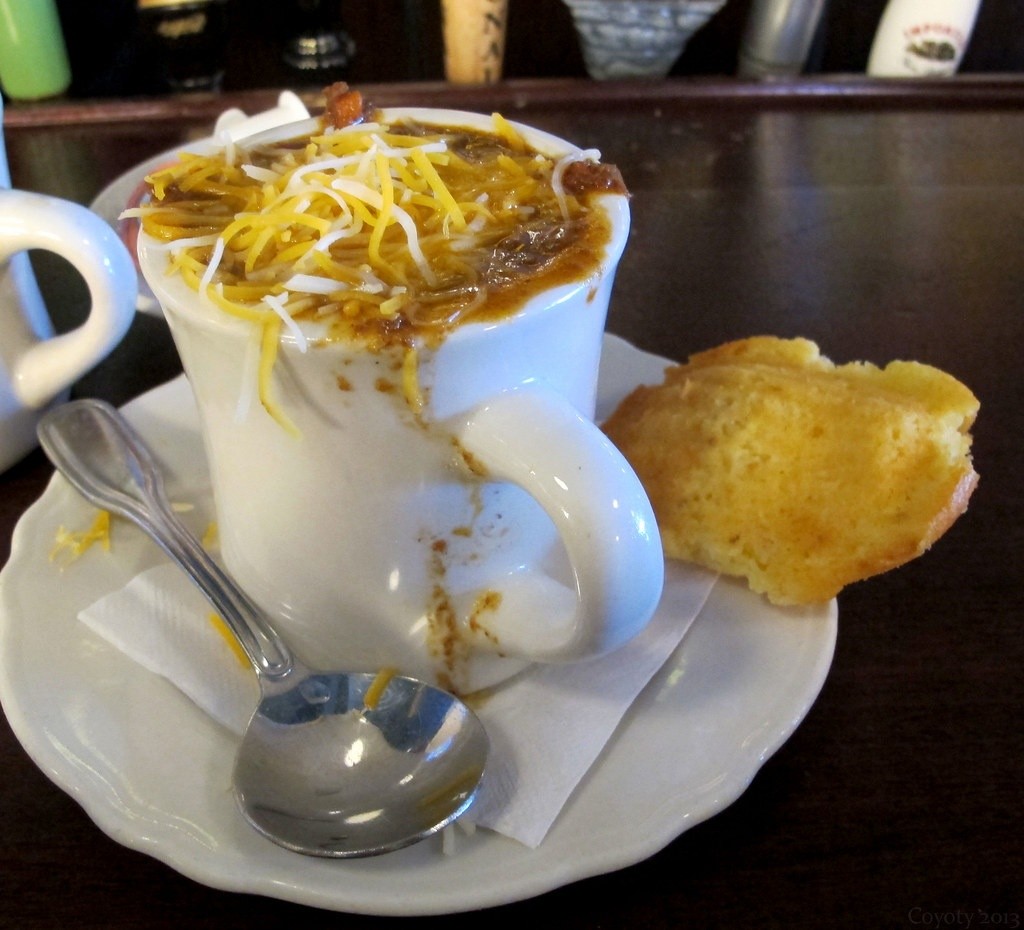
[597,332,981,610]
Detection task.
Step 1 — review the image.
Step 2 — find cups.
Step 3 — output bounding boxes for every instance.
[136,105,665,694]
[0,331,839,917]
[0,94,136,472]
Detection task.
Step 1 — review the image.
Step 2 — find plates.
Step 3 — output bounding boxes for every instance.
[87,107,633,320]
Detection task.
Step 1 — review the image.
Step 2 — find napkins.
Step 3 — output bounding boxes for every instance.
[76,536,722,855]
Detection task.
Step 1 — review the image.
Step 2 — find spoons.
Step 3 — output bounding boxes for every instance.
[37,398,490,858]
